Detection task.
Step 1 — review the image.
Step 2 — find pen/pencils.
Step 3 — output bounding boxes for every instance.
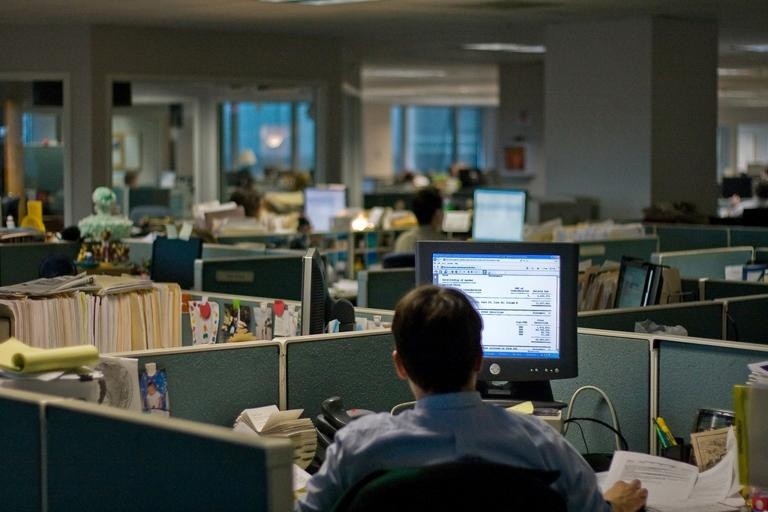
[653,417,678,448]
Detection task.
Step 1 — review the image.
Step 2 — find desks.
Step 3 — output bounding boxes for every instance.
[0,183,768,512]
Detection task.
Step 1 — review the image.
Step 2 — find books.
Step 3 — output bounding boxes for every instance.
[577,254,682,311]
[0,269,183,361]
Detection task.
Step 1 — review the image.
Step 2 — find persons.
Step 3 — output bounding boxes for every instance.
[291,285,648,512]
[392,188,445,256]
[736,181,768,218]
[145,381,164,409]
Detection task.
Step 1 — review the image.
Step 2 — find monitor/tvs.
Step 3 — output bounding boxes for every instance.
[414,238,580,409]
[300,247,355,338]
[302,183,349,231]
[613,254,665,307]
[472,188,529,240]
